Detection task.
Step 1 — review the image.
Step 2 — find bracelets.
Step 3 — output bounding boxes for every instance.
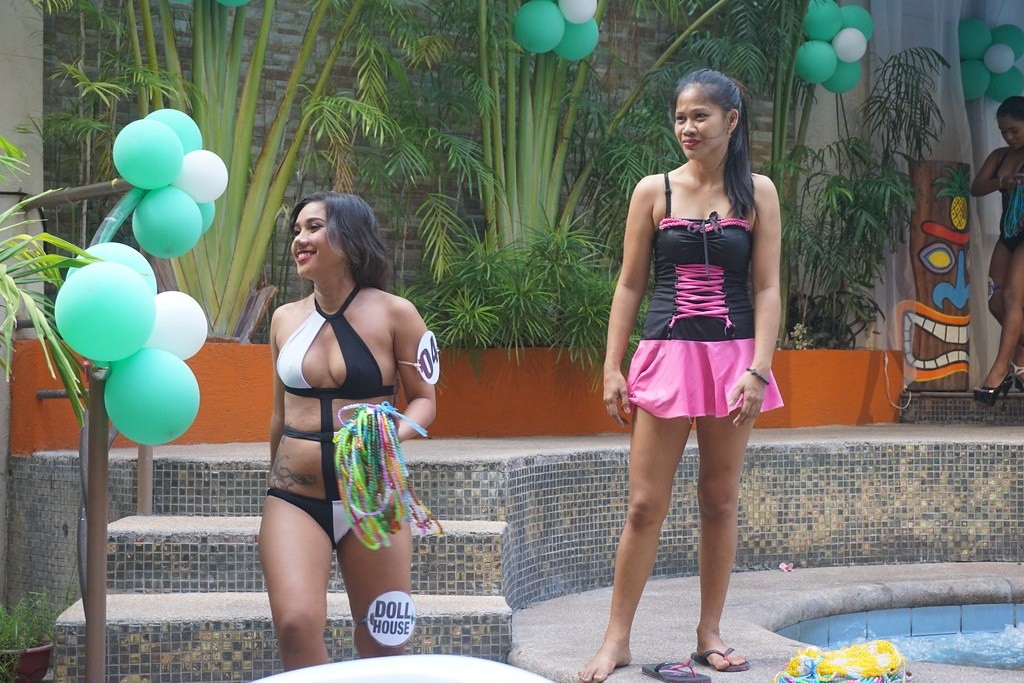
[747,368,769,385]
[1000,176,1003,189]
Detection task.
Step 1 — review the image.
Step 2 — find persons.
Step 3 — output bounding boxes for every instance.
[579,68,780,683]
[259,190,435,672]
[971,96,1024,406]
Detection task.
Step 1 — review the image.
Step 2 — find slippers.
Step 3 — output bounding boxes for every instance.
[691,647,750,672]
[642,660,711,683]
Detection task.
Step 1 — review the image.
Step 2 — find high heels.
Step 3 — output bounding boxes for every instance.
[973,370,1014,412]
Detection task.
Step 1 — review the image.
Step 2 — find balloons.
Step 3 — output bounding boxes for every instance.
[558,0,597,23]
[553,17,599,61]
[515,0,565,52]
[217,0,251,6]
[795,0,873,92]
[957,17,1024,103]
[54,109,228,444]
